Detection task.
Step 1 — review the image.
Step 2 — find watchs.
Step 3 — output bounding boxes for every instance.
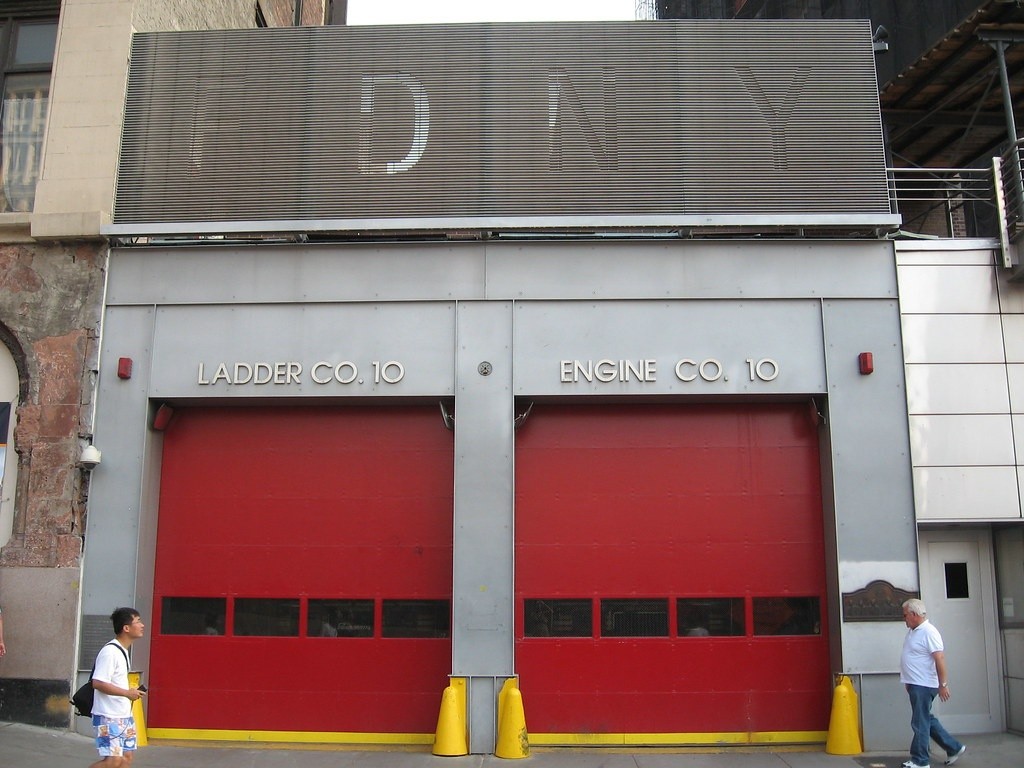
[939,682,948,688]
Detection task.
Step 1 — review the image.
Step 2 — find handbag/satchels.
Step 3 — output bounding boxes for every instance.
[70,682,94,718]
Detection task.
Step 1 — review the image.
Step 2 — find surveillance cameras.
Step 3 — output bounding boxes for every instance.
[80,445,102,470]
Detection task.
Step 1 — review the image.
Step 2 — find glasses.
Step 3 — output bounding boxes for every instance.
[119,619,142,627]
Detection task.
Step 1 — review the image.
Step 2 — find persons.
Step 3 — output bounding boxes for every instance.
[204,613,219,636]
[319,614,339,637]
[900,599,967,768]
[89,607,146,768]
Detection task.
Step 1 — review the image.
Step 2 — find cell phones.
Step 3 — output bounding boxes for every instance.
[137,684,147,695]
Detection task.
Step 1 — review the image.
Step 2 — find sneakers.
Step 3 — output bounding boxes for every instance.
[945,745,966,765]
[902,760,930,768]
